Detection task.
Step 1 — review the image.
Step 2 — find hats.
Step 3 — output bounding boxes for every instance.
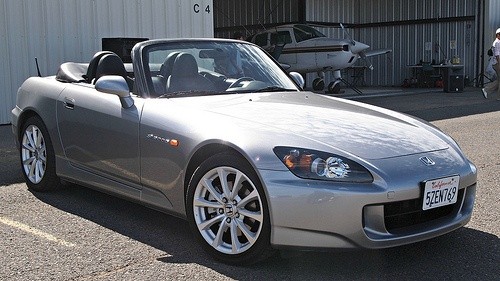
[496,28,500,34]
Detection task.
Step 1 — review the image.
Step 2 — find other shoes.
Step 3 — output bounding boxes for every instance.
[481,88,488,99]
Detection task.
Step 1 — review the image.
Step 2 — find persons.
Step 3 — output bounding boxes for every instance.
[234,32,243,40]
[481,27,500,99]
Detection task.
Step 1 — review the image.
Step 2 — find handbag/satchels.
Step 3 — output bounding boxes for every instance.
[488,49,493,56]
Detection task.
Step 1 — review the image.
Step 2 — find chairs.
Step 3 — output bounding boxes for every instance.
[86,52,215,93]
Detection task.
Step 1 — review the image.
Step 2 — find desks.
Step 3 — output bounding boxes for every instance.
[406,65,465,83]
[328,66,368,86]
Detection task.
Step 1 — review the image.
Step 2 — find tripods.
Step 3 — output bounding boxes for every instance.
[470,56,493,88]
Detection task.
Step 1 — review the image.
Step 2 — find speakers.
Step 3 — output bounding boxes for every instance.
[444,74,465,93]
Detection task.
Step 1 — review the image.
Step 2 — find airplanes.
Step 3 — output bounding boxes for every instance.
[242,22,393,95]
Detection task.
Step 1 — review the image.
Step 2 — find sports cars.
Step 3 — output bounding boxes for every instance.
[10,38,478,267]
